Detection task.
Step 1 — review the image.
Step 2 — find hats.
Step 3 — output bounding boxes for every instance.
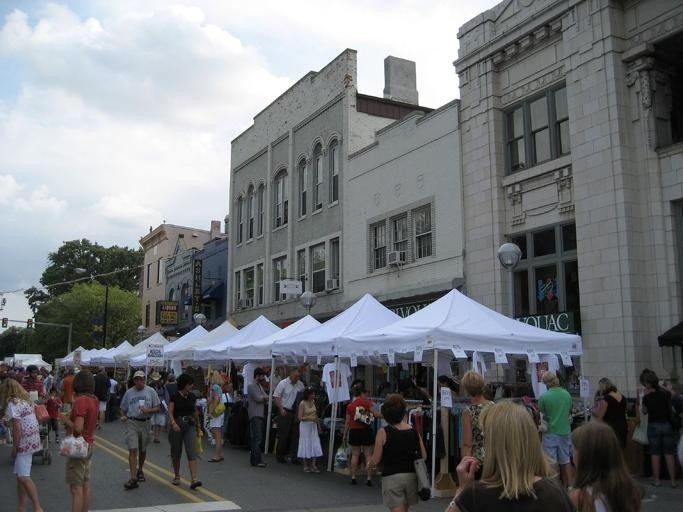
[133,371,146,379]
[149,372,162,381]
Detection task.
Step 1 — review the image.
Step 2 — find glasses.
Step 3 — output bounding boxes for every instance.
[309,385,312,390]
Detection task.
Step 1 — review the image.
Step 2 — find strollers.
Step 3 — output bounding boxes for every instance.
[11,400,52,467]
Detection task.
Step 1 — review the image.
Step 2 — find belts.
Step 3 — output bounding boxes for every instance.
[127,417,150,421]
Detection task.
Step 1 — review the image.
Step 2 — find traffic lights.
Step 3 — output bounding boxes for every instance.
[27,317,33,329]
[2,319,8,328]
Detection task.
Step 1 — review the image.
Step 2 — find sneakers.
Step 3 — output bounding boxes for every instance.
[276,457,288,463]
[48,439,52,443]
[292,458,301,465]
[303,465,311,472]
[256,463,266,467]
[56,439,59,443]
[310,465,321,473]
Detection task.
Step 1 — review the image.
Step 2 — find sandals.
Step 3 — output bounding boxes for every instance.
[137,470,145,482]
[189,479,202,489]
[124,476,139,488]
[173,476,180,485]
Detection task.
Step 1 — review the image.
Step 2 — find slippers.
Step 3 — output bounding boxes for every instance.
[208,458,224,462]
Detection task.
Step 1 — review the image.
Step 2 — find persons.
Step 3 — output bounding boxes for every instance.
[1,360,683,510]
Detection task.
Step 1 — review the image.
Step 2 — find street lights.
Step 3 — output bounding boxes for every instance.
[192,312,207,328]
[299,291,315,316]
[497,243,522,319]
[137,325,147,342]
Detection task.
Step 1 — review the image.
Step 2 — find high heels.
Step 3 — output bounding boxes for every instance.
[366,480,371,487]
[351,479,358,485]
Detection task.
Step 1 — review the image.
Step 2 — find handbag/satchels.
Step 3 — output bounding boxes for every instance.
[34,404,51,424]
[274,412,298,441]
[318,421,322,434]
[537,410,548,433]
[208,398,226,418]
[57,429,88,459]
[334,440,352,475]
[354,405,375,427]
[413,458,431,501]
